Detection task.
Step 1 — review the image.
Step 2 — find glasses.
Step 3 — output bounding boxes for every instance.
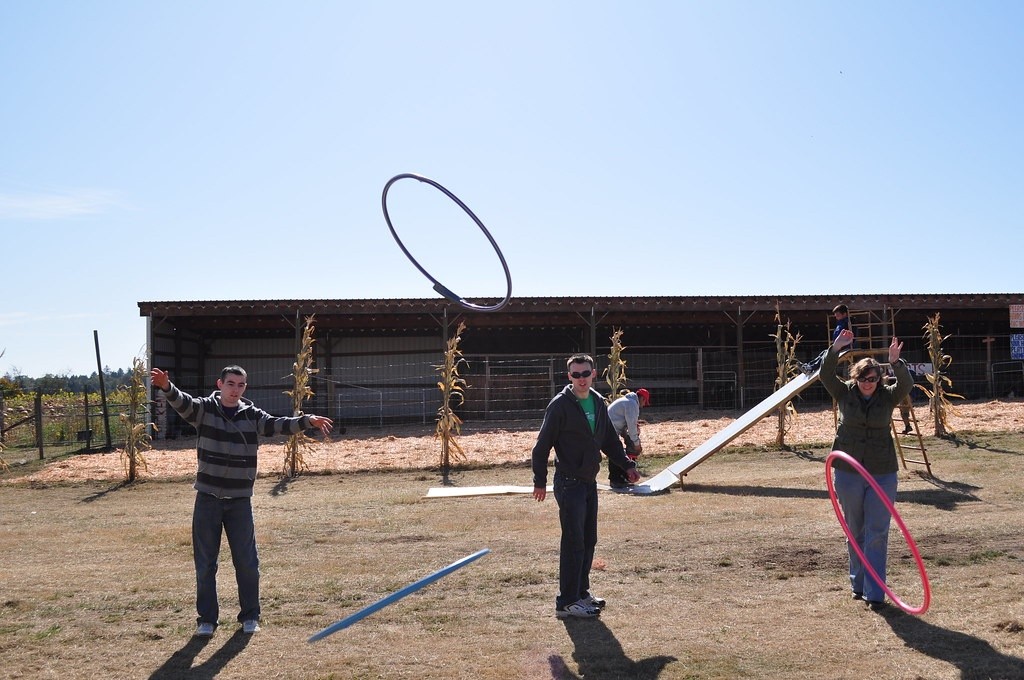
[570,370,592,378]
[857,376,878,382]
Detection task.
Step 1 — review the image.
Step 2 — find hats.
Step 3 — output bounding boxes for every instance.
[637,388,650,406]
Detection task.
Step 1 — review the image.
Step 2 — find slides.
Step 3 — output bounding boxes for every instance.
[668,348,853,478]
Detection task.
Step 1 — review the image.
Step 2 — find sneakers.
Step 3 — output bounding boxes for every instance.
[196,623,214,637]
[555,599,601,617]
[581,593,606,610]
[242,620,260,634]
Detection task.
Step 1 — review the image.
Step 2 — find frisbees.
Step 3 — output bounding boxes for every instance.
[308,547,489,644]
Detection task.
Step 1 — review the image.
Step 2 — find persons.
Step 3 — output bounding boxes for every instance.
[150,365,333,636]
[818,329,913,607]
[796,305,858,375]
[532,353,650,616]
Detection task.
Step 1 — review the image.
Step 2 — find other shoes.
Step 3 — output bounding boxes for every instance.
[852,592,863,600]
[796,361,812,376]
[610,481,634,488]
[867,601,885,610]
[902,425,912,434]
